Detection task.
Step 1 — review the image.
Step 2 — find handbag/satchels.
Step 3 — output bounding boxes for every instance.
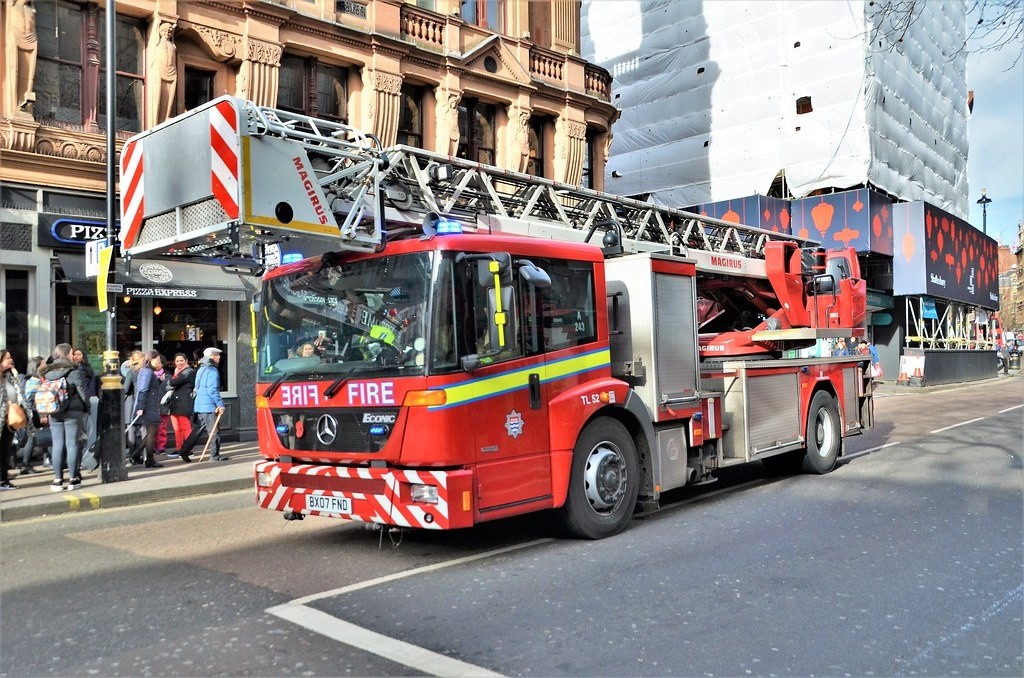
[6,401,28,433]
[159,399,173,415]
[35,425,52,446]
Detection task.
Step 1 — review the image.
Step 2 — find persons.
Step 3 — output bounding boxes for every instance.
[832,336,880,399]
[997,339,1014,374]
[0,341,102,492]
[293,295,352,365]
[119,348,230,467]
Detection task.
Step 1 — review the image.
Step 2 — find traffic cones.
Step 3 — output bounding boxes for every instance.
[896,353,911,381]
[911,351,924,378]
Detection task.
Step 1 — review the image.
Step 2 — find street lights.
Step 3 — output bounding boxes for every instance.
[974,188,993,235]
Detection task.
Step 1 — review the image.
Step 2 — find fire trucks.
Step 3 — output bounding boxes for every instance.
[118,94,877,542]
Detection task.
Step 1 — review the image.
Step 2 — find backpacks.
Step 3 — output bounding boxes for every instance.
[34,367,74,415]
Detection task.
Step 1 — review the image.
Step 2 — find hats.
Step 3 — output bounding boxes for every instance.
[203,347,222,357]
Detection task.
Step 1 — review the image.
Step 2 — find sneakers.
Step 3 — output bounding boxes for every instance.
[0,480,20,491]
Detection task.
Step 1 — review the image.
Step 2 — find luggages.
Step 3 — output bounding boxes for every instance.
[79,414,140,471]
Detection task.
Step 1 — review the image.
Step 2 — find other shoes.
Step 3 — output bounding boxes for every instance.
[51,479,65,491]
[129,453,143,464]
[177,450,191,462]
[210,455,228,461]
[1004,373,1009,375]
[146,458,163,468]
[68,477,81,490]
[20,465,38,473]
[179,452,194,458]
[167,451,180,457]
[153,451,165,455]
